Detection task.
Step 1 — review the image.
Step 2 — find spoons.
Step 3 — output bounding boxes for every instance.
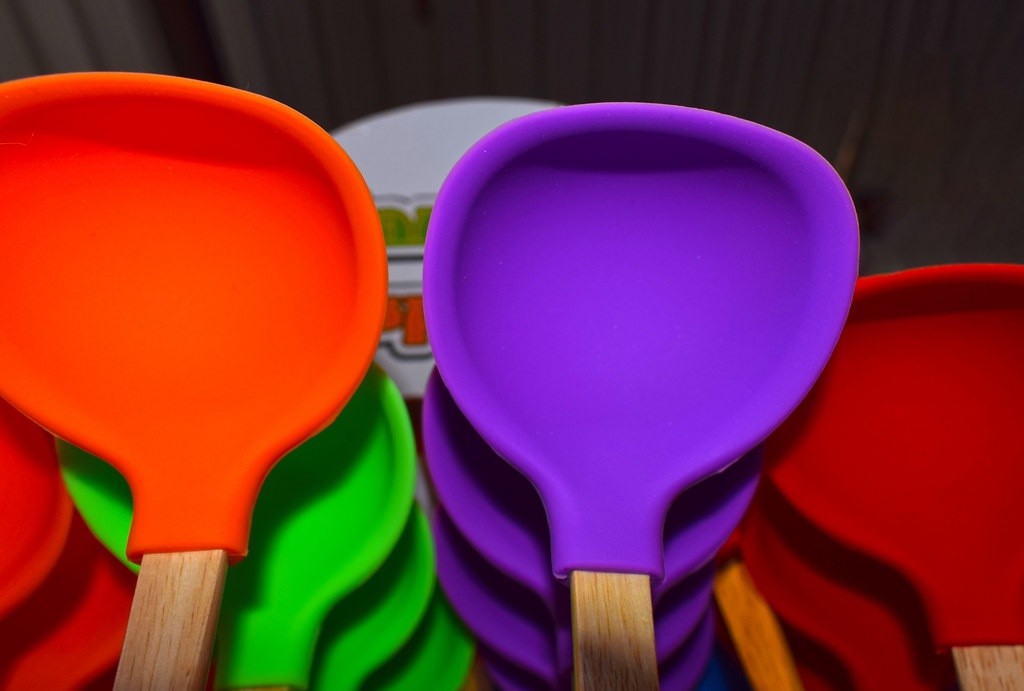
[0,72,1024,691]
